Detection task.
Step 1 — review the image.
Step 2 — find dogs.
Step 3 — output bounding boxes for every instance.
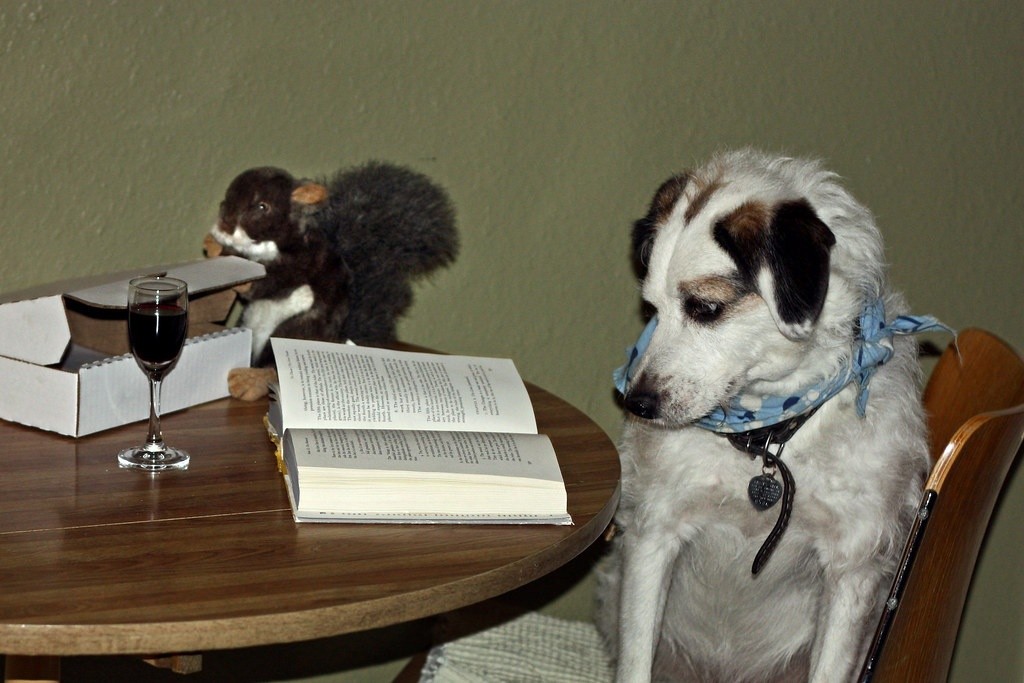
[592,143,930,683]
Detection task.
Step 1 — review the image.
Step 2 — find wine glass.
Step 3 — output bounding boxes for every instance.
[116,274,191,474]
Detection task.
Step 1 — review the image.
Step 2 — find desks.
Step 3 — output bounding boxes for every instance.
[1,342,623,683]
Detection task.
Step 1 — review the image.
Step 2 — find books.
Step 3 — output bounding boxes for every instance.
[263,337,574,523]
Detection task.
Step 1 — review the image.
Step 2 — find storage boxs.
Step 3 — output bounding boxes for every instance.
[0,254,267,436]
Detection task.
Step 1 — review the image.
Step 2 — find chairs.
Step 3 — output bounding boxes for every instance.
[393,329,1024,682]
